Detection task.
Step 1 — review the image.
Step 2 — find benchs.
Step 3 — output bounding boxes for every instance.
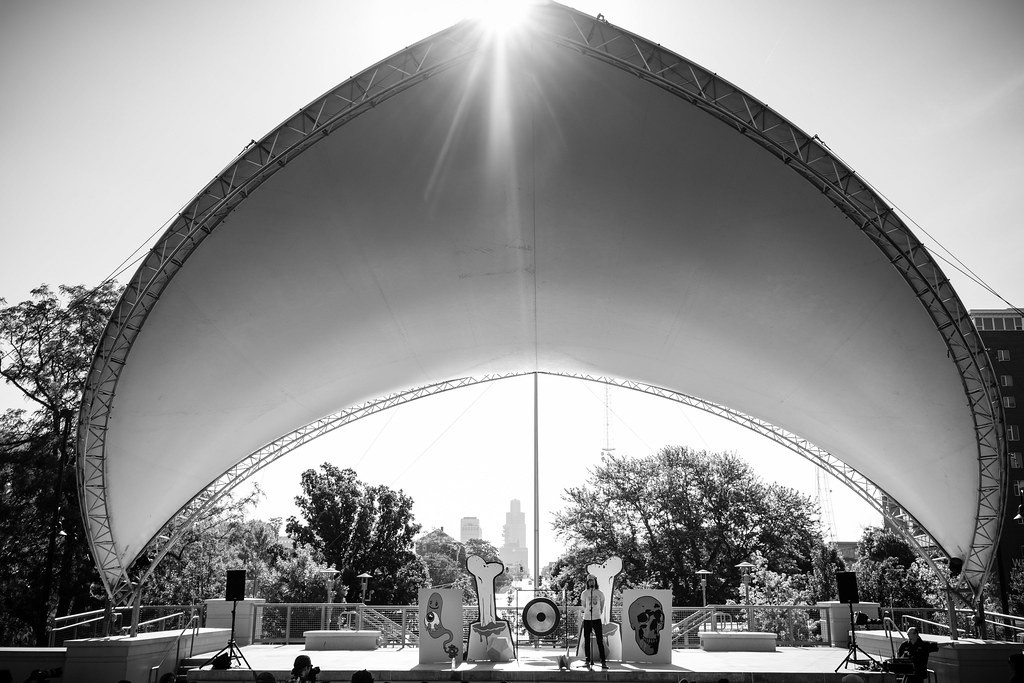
[303,630,381,651]
[697,631,778,652]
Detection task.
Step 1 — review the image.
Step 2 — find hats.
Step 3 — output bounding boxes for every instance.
[587,574,595,581]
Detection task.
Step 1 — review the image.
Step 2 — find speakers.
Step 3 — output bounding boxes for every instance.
[836,571,859,604]
[226,570,246,602]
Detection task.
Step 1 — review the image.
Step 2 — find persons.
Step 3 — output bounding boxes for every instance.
[898,626,929,683]
[289,655,320,683]
[581,574,609,669]
[159,673,176,683]
[351,670,375,683]
[256,672,275,683]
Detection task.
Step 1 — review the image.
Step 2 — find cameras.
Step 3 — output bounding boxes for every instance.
[306,665,320,682]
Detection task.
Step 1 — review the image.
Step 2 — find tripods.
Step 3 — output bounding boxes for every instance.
[576,589,610,671]
[199,601,252,669]
[835,604,884,673]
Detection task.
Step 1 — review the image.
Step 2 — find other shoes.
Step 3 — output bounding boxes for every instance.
[582,661,590,667]
[602,663,609,669]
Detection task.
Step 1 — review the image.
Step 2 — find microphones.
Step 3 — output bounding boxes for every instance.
[590,585,594,589]
